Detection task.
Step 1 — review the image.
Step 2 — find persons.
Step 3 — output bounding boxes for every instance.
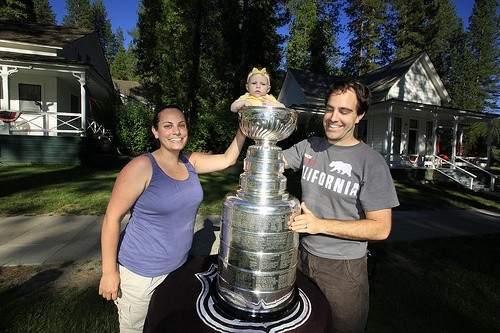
[101,105,246,333]
[282,80,392,333]
[230,67,286,113]
